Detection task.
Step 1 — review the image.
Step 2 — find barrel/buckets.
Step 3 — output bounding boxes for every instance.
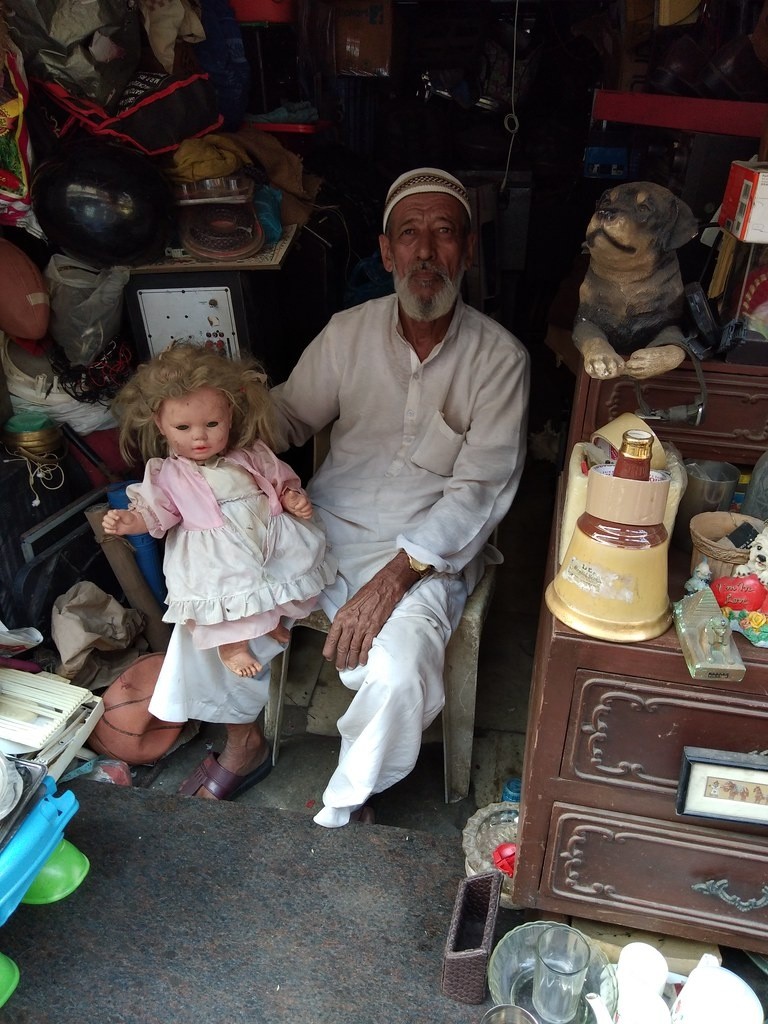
[677,460,742,530]
[691,512,766,584]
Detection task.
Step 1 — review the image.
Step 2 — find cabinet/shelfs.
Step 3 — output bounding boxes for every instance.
[508,344,767,958]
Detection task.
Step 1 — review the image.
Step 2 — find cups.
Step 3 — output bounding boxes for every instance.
[481,1005,538,1024]
[533,925,592,1023]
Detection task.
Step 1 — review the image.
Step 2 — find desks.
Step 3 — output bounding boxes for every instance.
[121,223,298,365]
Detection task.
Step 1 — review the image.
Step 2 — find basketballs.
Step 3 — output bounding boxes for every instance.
[86,651,184,763]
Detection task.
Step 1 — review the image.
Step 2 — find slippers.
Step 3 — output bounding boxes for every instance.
[177,736,276,799]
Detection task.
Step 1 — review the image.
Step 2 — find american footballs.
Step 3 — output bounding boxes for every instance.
[0,239,50,341]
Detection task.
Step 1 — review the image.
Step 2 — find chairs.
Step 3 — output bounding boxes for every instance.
[263,420,499,804]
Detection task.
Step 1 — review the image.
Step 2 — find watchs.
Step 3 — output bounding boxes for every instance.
[400,549,432,579]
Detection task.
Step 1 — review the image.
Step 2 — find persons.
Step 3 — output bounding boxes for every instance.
[100,336,340,677]
[149,166,534,828]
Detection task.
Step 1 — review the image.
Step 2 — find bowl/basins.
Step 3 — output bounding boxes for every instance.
[462,801,520,893]
[487,920,618,1024]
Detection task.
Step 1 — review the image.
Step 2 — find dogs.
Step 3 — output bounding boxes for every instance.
[570,181,699,380]
[735,534,768,589]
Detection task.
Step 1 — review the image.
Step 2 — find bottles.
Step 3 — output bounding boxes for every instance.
[503,776,521,802]
[545,429,674,642]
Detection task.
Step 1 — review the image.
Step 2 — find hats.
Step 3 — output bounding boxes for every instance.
[382,167,471,235]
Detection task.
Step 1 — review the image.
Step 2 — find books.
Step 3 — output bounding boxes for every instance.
[698,230,737,302]
